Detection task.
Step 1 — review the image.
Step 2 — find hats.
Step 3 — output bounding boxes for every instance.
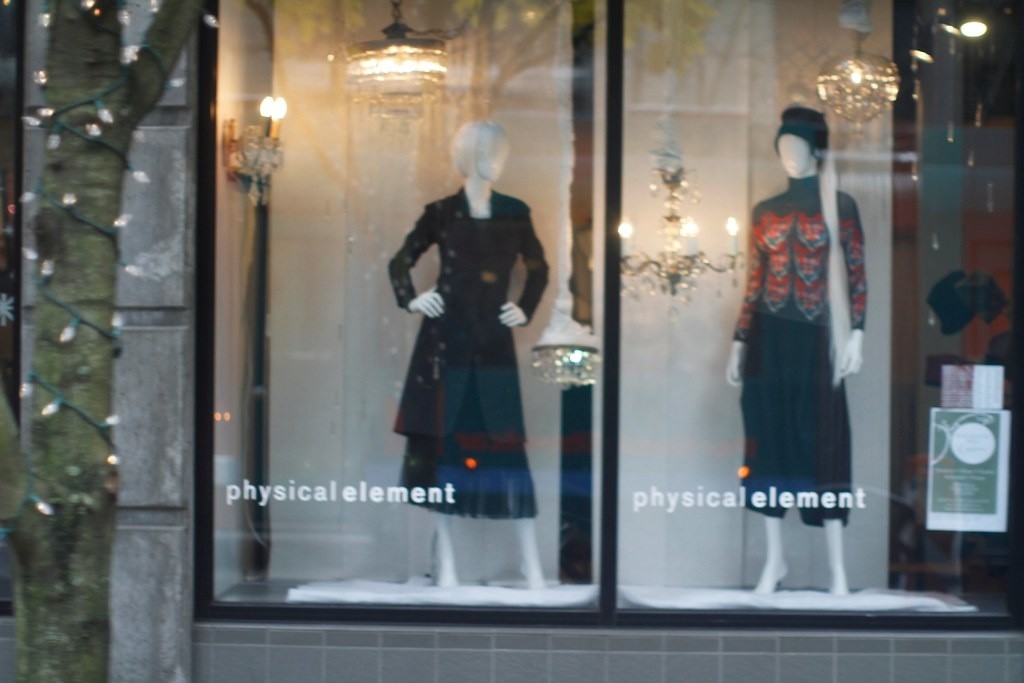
[954,268,1008,325]
[926,271,978,337]
[776,107,828,149]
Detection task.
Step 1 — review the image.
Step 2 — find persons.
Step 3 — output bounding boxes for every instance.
[386,121,549,593]
[722,108,866,602]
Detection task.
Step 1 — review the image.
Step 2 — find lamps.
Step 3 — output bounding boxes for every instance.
[532,1,599,387]
[222,95,289,206]
[347,0,449,114]
[816,6,901,135]
[619,166,744,298]
